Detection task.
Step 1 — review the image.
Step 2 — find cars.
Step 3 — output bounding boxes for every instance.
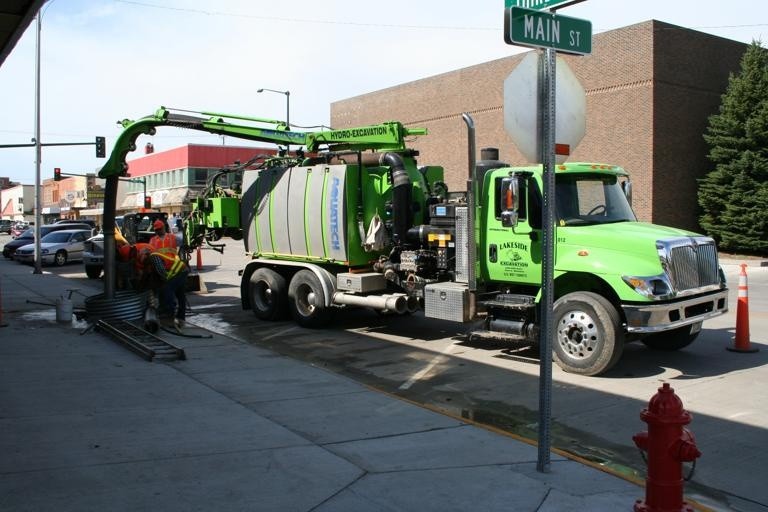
[0,216,181,280]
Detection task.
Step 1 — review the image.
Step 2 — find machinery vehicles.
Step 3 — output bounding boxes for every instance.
[98,106,729,376]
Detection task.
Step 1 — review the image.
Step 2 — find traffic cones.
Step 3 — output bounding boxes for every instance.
[726,263,760,353]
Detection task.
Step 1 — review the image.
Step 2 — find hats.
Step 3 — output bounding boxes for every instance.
[139,246,151,255]
[153,220,164,230]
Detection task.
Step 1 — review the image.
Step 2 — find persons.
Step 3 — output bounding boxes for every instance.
[148,218,188,255]
[119,242,159,291]
[135,249,191,327]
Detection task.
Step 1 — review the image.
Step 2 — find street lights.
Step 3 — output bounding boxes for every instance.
[257,89,290,126]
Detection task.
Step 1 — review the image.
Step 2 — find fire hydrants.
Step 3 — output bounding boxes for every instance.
[632,383,702,512]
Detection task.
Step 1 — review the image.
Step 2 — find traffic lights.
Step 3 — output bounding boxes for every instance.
[54,168,60,181]
[145,197,151,209]
[96,136,105,158]
